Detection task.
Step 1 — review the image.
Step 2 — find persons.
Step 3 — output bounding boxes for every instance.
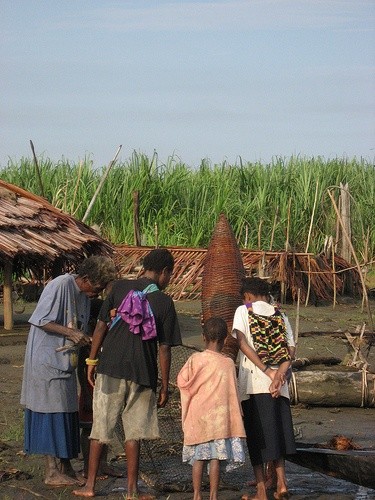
[77,298,124,481]
[73,246,184,500]
[177,317,248,500]
[230,276,299,500]
[20,255,117,486]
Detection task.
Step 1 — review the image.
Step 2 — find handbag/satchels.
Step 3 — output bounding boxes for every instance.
[246,302,291,365]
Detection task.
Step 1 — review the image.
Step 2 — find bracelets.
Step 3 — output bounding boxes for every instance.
[261,364,271,372]
[84,356,99,366]
